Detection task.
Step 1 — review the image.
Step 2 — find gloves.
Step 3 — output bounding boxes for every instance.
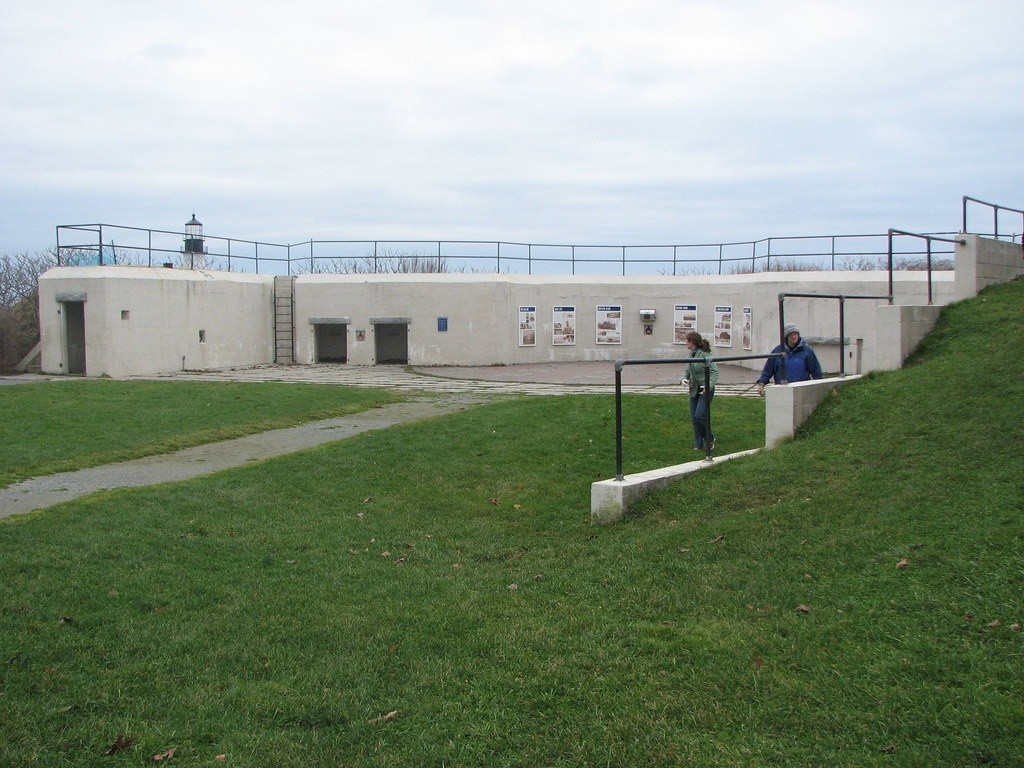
[757,383,765,396]
[681,378,689,388]
[698,386,705,395]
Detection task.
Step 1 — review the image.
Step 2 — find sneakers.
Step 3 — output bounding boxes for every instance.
[710,437,716,450]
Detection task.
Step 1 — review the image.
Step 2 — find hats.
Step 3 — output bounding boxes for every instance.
[784,323,800,337]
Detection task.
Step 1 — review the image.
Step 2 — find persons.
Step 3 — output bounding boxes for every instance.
[756,324,824,396]
[680,332,719,450]
